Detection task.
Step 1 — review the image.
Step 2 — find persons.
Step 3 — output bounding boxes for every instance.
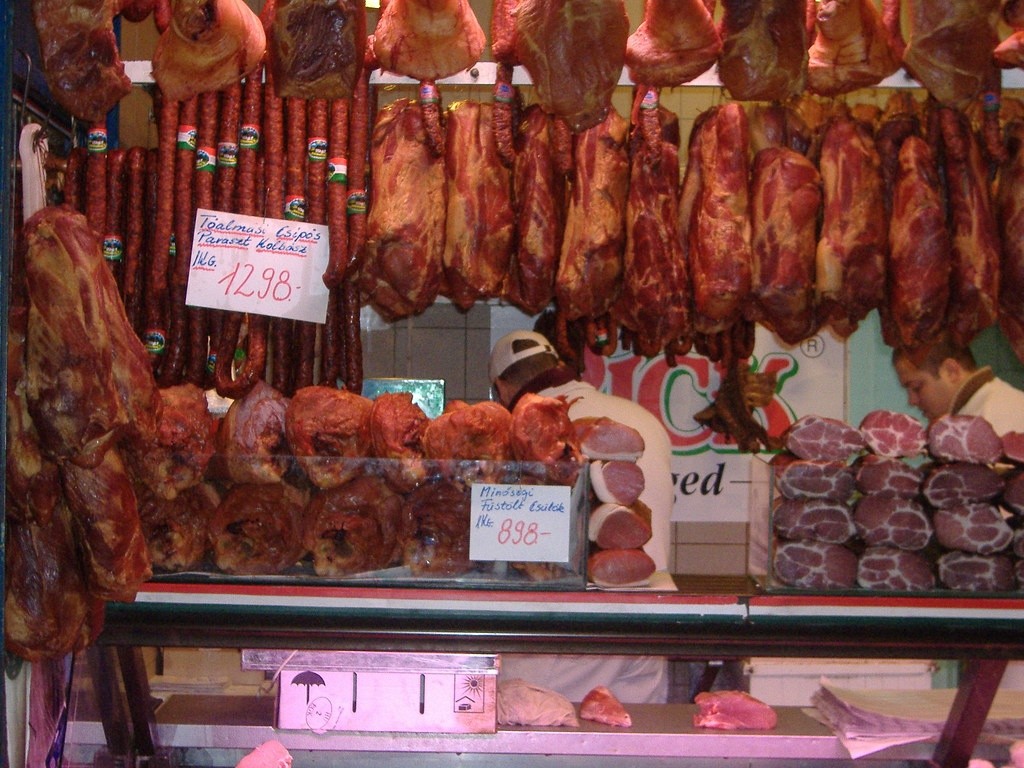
[488,332,674,702]
[891,342,1024,437]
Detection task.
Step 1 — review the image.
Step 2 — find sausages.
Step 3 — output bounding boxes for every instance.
[551,296,758,370]
[936,66,1011,167]
[65,0,570,400]
[631,82,663,166]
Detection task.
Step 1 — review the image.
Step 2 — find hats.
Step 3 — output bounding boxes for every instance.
[486,332,559,400]
